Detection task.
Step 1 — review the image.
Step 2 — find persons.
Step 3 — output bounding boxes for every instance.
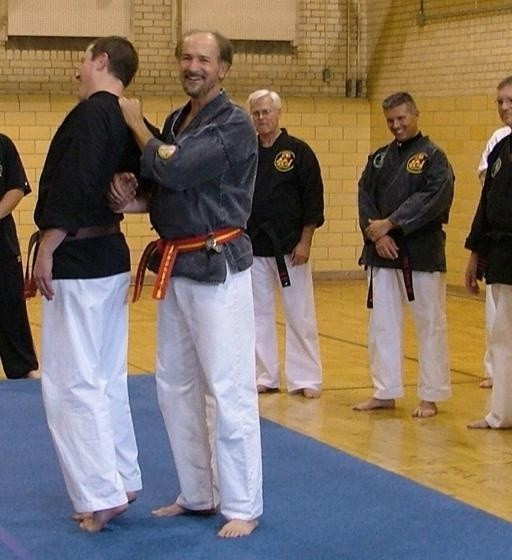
[353,90,455,418]
[0,134,42,380]
[465,76,512,429]
[108,29,263,538]
[32,36,161,533]
[245,89,325,398]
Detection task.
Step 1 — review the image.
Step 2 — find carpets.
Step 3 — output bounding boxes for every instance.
[2,374,512,560]
